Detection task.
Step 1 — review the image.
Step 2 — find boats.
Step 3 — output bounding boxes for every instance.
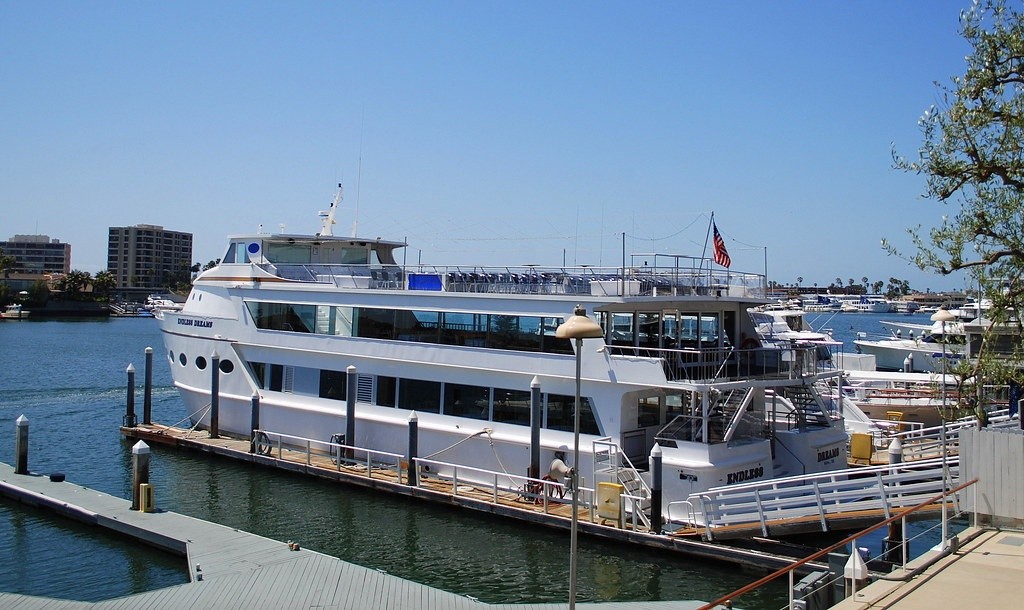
[152,212,851,540]
[840,304,859,313]
[145,294,183,311]
[947,299,1016,322]
[804,304,833,312]
[853,326,966,376]
[878,320,969,339]
[1,304,31,319]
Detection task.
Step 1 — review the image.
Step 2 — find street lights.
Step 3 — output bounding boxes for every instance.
[930,307,957,548]
[556,309,603,610]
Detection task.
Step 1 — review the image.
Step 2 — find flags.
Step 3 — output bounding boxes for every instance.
[714,220,732,268]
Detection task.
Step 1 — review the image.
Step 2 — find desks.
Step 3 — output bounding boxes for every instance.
[876,388,938,397]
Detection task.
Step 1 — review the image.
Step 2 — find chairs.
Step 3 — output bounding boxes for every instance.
[446,271,708,295]
